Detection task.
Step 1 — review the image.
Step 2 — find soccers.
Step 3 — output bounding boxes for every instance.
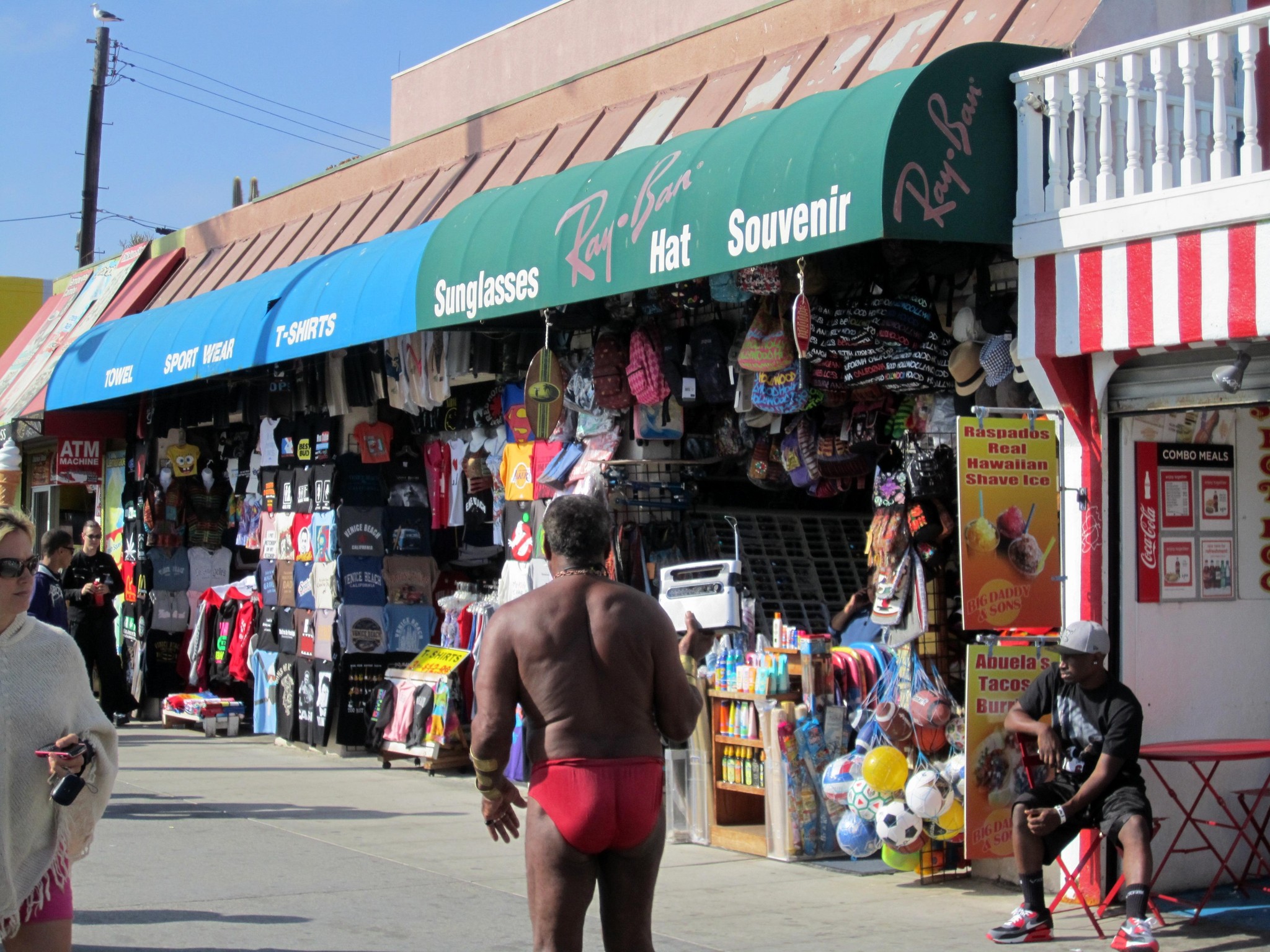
[945,717,965,750]
[821,745,964,858]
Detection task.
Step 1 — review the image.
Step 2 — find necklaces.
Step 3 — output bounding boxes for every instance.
[553,562,609,578]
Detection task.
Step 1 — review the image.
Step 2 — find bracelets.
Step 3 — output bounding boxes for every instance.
[469,744,511,800]
[679,655,698,687]
[1054,805,1066,824]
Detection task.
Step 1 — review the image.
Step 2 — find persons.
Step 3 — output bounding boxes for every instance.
[827,570,884,648]
[471,494,715,952]
[63,520,125,724]
[27,529,74,631]
[987,620,1159,952]
[0,510,118,952]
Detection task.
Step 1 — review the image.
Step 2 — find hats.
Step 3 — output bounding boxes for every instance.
[948,340,987,396]
[952,305,992,346]
[983,291,1018,335]
[979,332,1015,388]
[1044,620,1111,656]
[1009,335,1028,383]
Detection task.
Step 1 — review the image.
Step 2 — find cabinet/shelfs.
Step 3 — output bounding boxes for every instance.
[705,688,797,857]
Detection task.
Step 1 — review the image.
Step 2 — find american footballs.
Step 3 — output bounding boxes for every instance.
[848,709,874,733]
[891,733,913,759]
[908,688,952,728]
[875,701,914,742]
[855,719,883,755]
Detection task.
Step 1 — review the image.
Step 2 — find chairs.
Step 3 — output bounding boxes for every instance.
[1231,787,1270,891]
[1013,732,1167,941]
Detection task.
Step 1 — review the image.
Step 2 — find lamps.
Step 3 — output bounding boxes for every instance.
[1210,349,1252,394]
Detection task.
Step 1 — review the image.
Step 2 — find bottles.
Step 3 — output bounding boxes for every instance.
[715,647,743,692]
[1144,471,1151,499]
[1175,557,1188,580]
[1202,559,1231,589]
[722,744,766,789]
[104,573,111,585]
[93,578,104,606]
[1213,489,1227,514]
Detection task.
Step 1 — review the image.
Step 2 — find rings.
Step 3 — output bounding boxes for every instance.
[500,813,506,818]
[485,819,493,825]
[494,818,500,823]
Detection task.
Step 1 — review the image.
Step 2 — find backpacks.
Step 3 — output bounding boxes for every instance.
[626,316,672,428]
[590,317,640,411]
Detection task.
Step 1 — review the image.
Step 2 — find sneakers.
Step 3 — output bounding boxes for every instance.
[986,902,1055,944]
[1109,916,1160,952]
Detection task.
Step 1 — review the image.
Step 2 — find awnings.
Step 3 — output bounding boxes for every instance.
[44,216,443,412]
[417,40,1072,332]
[0,247,185,447]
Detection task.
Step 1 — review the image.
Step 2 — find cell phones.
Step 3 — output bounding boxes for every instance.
[35,743,87,760]
[1037,749,1066,770]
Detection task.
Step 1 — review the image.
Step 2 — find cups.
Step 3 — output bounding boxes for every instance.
[964,511,1045,579]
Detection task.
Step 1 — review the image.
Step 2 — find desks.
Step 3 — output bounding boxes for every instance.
[1133,737,1270,929]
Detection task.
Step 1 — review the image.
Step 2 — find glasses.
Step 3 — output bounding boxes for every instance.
[83,533,101,539]
[0,555,39,578]
[61,546,75,555]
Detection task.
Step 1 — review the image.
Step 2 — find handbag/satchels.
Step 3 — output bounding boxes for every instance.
[670,255,960,650]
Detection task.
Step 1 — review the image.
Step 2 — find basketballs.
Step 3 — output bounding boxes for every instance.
[912,723,947,753]
